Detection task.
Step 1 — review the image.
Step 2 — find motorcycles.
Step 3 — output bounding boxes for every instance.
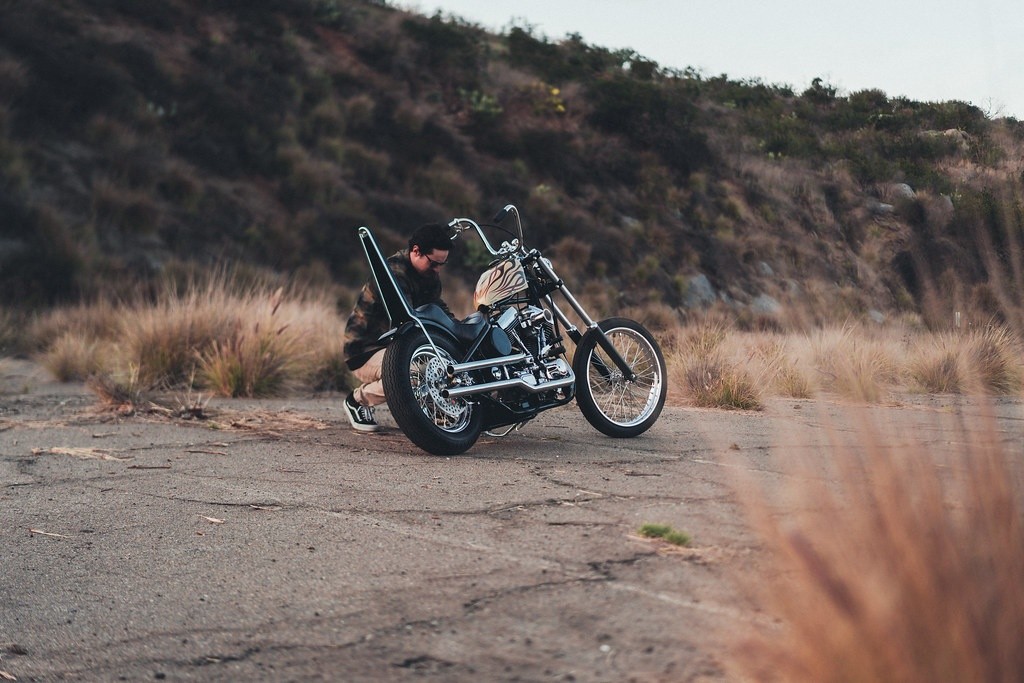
[358,204,667,457]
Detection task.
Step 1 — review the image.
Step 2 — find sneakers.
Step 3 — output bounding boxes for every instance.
[343,391,378,431]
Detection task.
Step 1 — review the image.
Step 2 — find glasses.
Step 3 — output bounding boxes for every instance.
[419,247,450,268]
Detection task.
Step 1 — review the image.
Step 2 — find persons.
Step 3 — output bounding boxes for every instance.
[342,224,460,433]
